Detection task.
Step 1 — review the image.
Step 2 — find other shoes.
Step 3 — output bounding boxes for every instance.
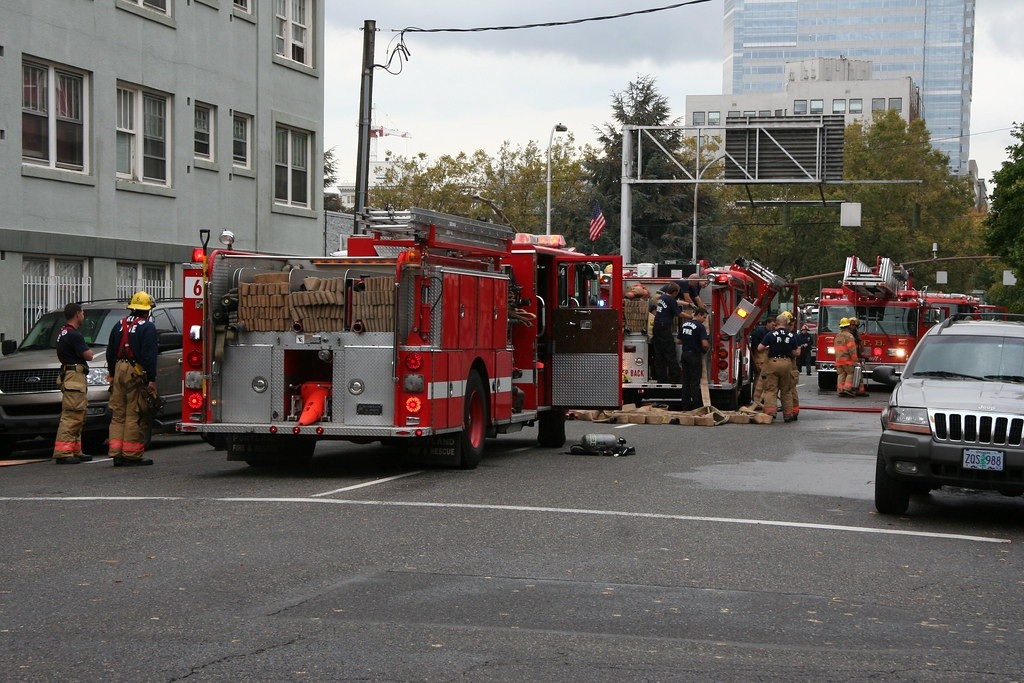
[56,456,81,464]
[112,457,153,467]
[844,390,856,397]
[838,393,848,397]
[74,454,93,461]
[856,393,869,397]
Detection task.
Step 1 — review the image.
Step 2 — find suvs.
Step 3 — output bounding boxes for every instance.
[0,296,187,452]
[874,312,1024,515]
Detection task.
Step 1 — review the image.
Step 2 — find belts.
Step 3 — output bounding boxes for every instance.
[61,365,89,375]
[768,355,789,359]
[656,290,663,295]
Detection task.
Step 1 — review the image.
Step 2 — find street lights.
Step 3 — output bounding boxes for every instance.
[545,122,568,238]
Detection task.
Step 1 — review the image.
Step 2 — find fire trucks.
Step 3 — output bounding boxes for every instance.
[815,287,1008,370]
[811,256,951,391]
[174,202,625,472]
[595,252,800,408]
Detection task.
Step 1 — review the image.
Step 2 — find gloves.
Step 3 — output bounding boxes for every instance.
[865,357,869,363]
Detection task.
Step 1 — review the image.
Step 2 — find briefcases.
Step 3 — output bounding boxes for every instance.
[852,366,861,388]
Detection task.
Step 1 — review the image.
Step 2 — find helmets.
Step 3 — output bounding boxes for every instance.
[838,317,851,327]
[801,325,810,332]
[849,316,860,326]
[127,290,156,310]
[779,311,797,325]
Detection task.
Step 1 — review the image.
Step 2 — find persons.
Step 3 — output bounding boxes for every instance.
[677,307,709,412]
[834,317,870,398]
[648,274,711,384]
[105,291,158,467]
[52,302,94,464]
[751,311,814,423]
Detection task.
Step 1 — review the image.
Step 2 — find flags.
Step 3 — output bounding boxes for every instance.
[589,200,606,242]
